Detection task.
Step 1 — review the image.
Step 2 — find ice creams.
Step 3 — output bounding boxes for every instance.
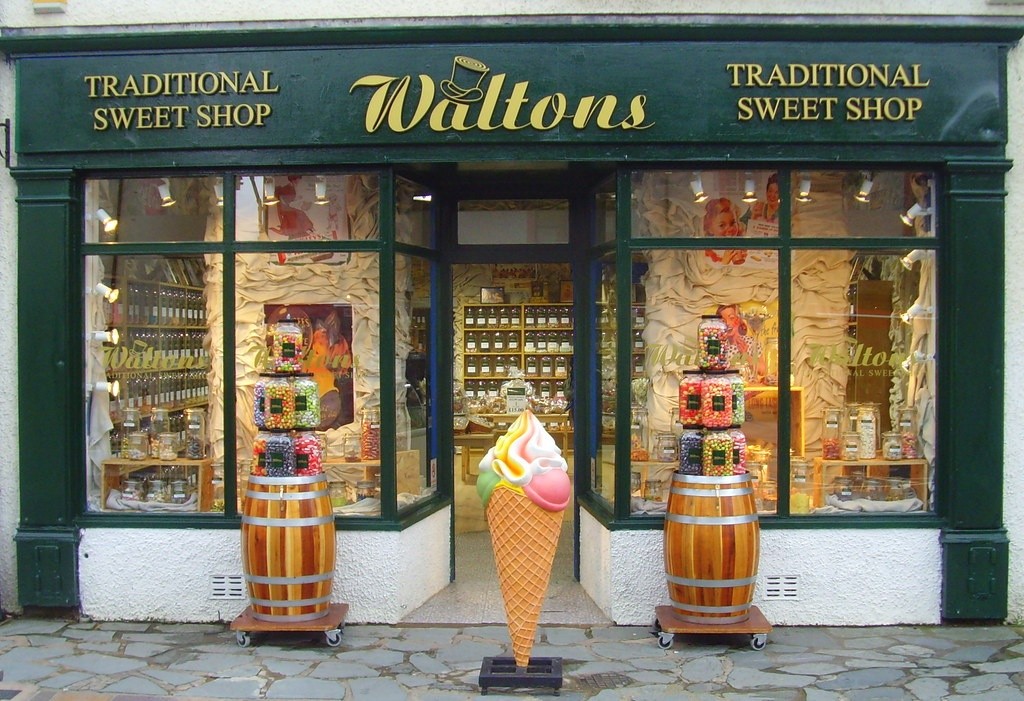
[477,411,572,667]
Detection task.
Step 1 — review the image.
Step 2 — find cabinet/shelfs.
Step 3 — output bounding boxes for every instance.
[461,303,646,408]
[102,273,211,425]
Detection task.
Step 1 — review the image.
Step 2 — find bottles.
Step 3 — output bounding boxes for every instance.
[250,317,324,477]
[113,282,207,327]
[524,305,570,399]
[118,479,189,506]
[747,445,815,513]
[464,306,520,397]
[108,370,209,415]
[820,401,880,461]
[658,313,747,474]
[120,408,205,460]
[882,406,918,459]
[829,471,911,502]
[119,328,209,366]
[630,407,650,462]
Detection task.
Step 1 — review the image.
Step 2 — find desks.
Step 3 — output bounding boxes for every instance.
[454,413,574,485]
[812,454,930,513]
[100,456,214,512]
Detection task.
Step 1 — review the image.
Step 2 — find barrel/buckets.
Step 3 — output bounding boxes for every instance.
[239,473,338,623]
[663,469,761,626]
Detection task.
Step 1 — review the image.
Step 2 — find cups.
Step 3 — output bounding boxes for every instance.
[357,479,375,504]
[327,480,347,507]
[342,410,381,462]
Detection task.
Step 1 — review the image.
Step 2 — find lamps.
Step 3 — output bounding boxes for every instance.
[95,328,120,346]
[903,350,923,372]
[742,180,758,203]
[691,179,709,204]
[96,380,120,396]
[264,178,279,206]
[314,181,330,206]
[214,183,224,206]
[899,249,922,271]
[900,303,923,326]
[899,202,921,226]
[158,184,176,208]
[795,180,812,203]
[854,179,873,203]
[96,209,118,233]
[96,282,120,305]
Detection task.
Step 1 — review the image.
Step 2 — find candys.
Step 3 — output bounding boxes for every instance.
[680,320,746,477]
[253,323,323,478]
